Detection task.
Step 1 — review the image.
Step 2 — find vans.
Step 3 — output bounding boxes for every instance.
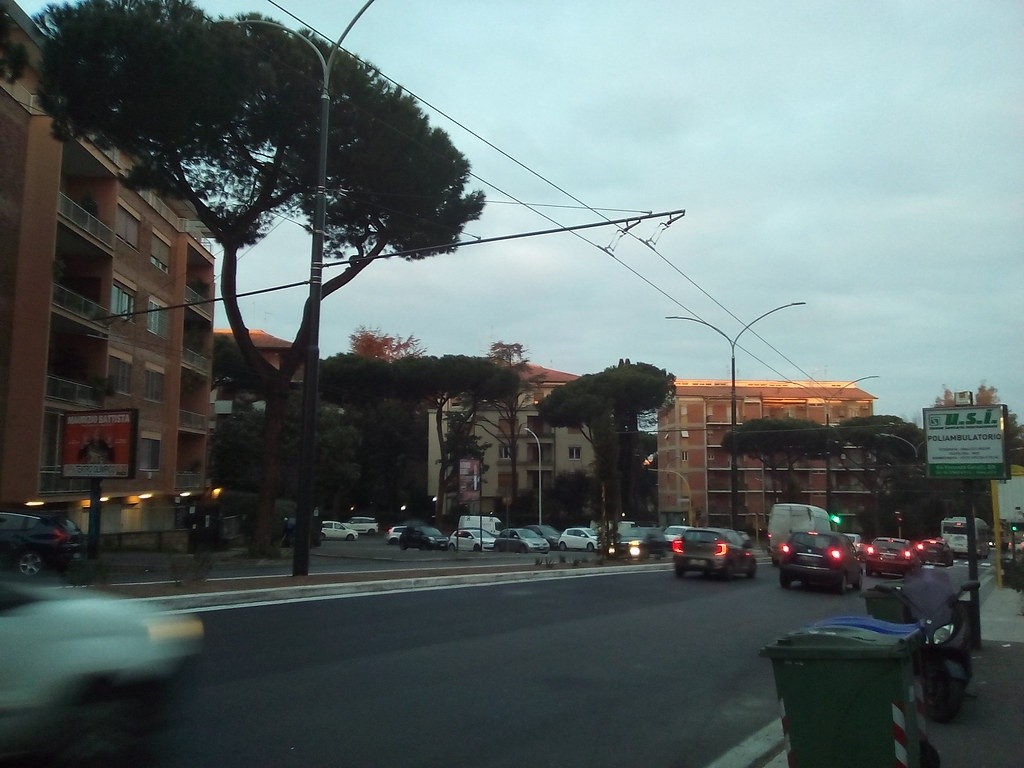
[458,515,504,534]
[766,503,835,565]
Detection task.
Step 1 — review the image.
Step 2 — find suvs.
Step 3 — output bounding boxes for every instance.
[0,507,88,578]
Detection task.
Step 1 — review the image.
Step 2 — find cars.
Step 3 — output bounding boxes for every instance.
[779,530,864,593]
[558,529,604,551]
[387,525,448,551]
[321,520,358,541]
[866,538,919,576]
[526,524,560,547]
[918,541,953,567]
[449,530,499,552]
[672,528,755,581]
[662,525,699,549]
[493,528,549,554]
[1008,538,1024,552]
[343,517,379,536]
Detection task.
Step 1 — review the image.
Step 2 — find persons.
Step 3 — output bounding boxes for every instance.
[77,428,116,464]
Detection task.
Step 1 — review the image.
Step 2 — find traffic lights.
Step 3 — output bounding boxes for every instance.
[831,516,840,524]
[898,517,904,525]
[1012,524,1017,531]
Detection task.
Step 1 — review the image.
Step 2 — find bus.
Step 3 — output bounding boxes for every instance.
[941,517,990,559]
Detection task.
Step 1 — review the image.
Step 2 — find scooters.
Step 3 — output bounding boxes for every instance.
[876,567,980,723]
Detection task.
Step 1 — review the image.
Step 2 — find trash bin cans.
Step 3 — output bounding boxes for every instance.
[759,617,944,768]
[862,579,913,623]
[1001,558,1018,587]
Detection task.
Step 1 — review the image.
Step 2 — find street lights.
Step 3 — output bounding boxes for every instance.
[778,375,880,511]
[524,427,541,525]
[665,302,807,527]
